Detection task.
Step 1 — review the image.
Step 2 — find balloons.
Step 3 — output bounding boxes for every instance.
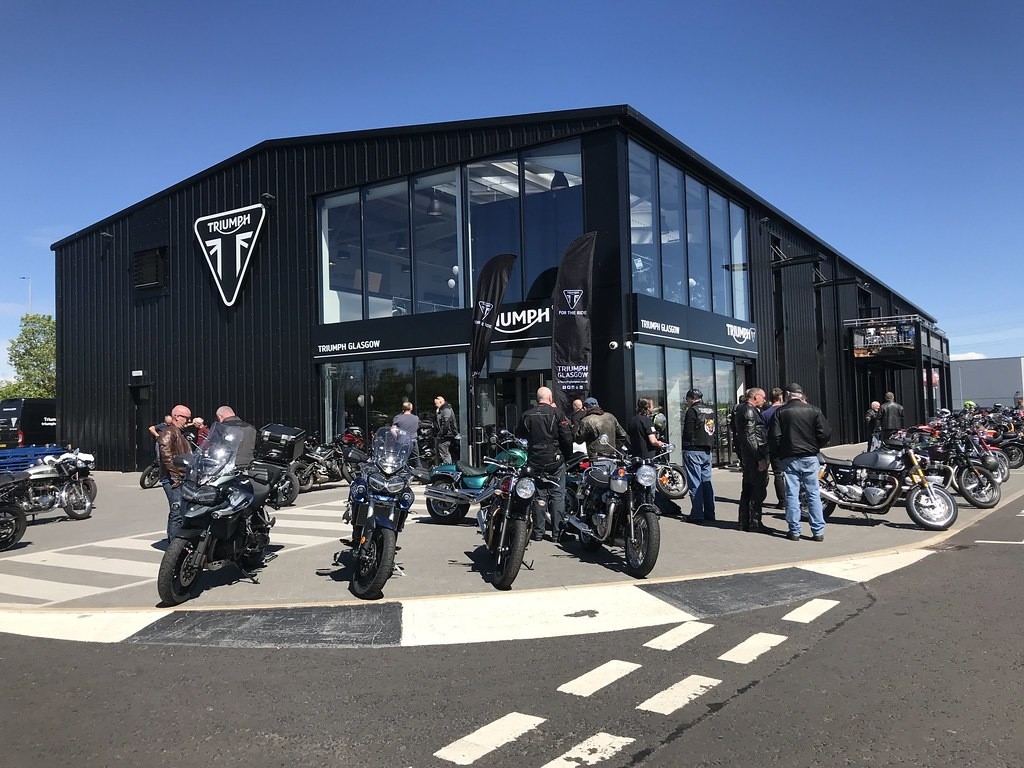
[357,394,374,408]
[401,396,409,403]
[448,266,458,288]
[405,383,413,394]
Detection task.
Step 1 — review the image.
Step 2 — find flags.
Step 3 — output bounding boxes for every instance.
[509,267,558,371]
[466,254,518,393]
[549,233,598,425]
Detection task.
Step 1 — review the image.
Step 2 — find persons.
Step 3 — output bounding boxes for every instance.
[149,415,210,461]
[1013,400,1024,422]
[730,387,769,534]
[962,401,976,417]
[680,389,716,524]
[158,405,193,546]
[637,266,654,294]
[498,421,505,442]
[864,401,882,453]
[760,383,832,541]
[345,412,354,429]
[1014,391,1023,407]
[675,278,702,305]
[550,399,587,454]
[515,387,574,543]
[208,406,256,477]
[392,401,423,481]
[432,395,458,464]
[626,396,666,520]
[875,392,904,448]
[570,398,627,461]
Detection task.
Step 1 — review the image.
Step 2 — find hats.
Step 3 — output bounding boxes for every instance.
[582,398,598,409]
[779,383,802,395]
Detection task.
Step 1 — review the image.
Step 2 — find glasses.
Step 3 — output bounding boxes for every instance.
[757,393,767,400]
[177,414,193,423]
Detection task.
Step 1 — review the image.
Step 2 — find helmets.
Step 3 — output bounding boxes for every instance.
[686,389,703,401]
[964,400,976,411]
[940,408,951,419]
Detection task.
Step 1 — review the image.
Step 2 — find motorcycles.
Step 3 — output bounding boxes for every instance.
[266,428,369,506]
[656,435,688,499]
[342,427,434,600]
[423,431,528,525]
[0,471,32,552]
[800,426,959,531]
[545,433,675,578]
[140,460,159,489]
[874,400,1024,509]
[157,422,307,606]
[476,433,564,590]
[16,448,93,525]
[42,441,98,509]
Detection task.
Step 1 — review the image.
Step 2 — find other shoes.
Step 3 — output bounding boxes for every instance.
[787,532,799,541]
[775,504,787,510]
[814,534,824,542]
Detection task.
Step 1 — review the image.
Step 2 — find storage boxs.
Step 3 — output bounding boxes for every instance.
[255,422,306,464]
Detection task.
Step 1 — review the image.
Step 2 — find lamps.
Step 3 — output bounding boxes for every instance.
[427,185,444,216]
[551,170,569,190]
[395,217,407,250]
[659,213,670,234]
[401,260,412,273]
[132,371,143,377]
[337,248,351,260]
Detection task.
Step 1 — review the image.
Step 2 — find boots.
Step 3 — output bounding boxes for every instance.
[738,496,776,533]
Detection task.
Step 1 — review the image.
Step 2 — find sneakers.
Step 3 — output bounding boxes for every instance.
[681,511,716,526]
[552,533,576,543]
[530,534,543,540]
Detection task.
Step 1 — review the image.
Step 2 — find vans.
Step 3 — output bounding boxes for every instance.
[0,398,56,449]
